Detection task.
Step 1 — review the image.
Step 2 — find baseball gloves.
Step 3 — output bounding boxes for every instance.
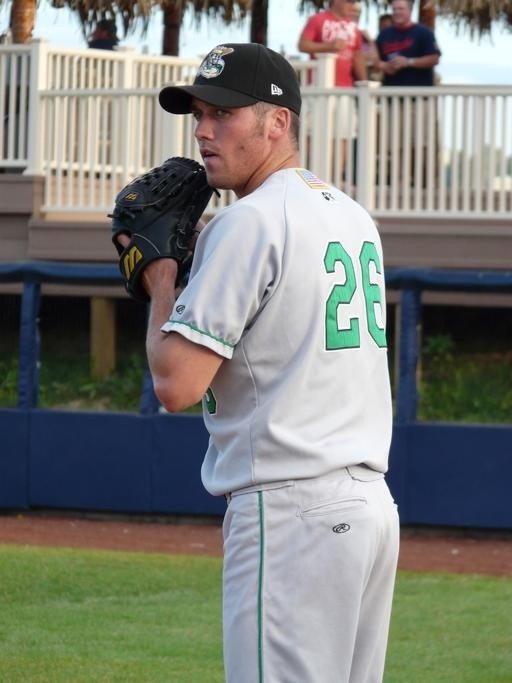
[107,156,217,302]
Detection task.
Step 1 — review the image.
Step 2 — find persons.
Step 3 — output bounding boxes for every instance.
[88,18,120,50]
[300,0,442,187]
[112,43,401,683]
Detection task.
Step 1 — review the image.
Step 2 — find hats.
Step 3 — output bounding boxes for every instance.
[159,43,301,115]
